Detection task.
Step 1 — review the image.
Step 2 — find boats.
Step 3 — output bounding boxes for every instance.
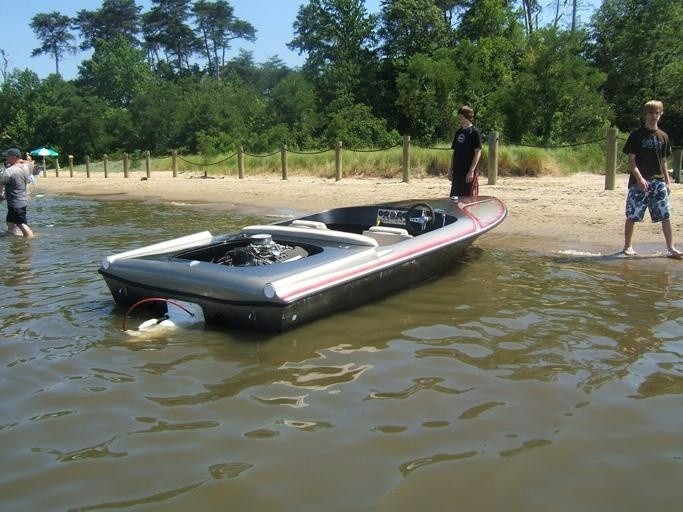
[89,190,508,349]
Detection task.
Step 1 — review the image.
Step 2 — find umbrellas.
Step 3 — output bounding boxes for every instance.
[30,148,59,176]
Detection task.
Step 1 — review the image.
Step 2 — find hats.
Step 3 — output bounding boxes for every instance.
[1,148,21,157]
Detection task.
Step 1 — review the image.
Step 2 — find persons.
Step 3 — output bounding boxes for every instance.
[448,105,482,197]
[0,148,34,238]
[622,100,683,259]
